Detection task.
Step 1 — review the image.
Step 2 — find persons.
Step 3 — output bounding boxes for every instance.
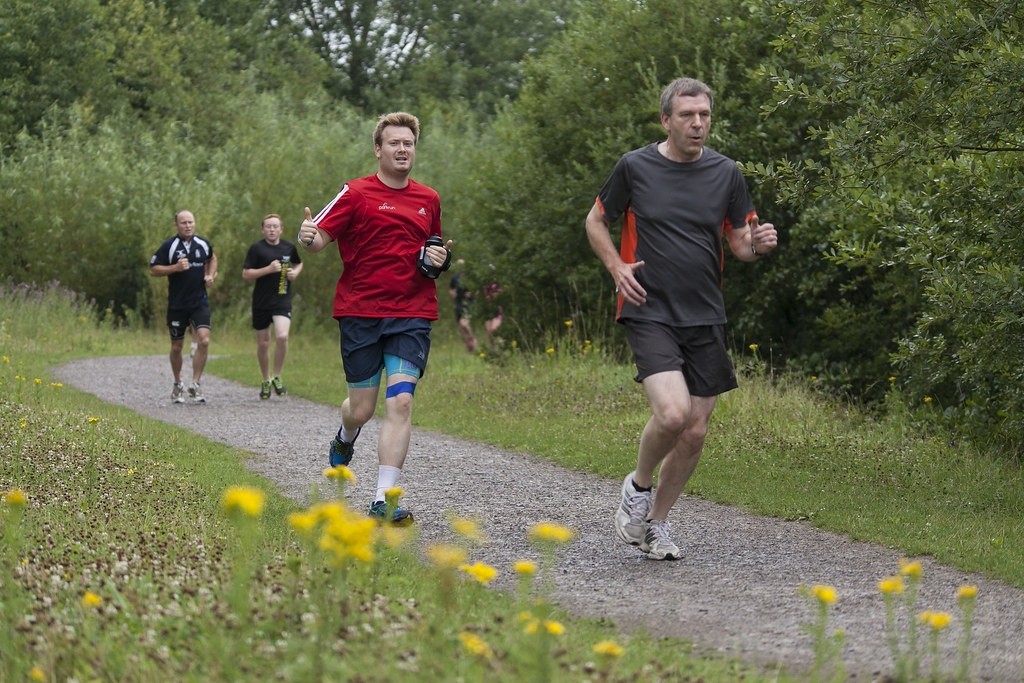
[150,209,217,404]
[448,257,505,352]
[242,214,304,399]
[584,77,777,560]
[297,112,453,528]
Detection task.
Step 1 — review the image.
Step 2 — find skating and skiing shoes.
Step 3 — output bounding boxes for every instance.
[367,501,414,527]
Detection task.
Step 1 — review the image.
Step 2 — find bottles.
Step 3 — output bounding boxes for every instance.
[274,259,289,294]
[421,231,444,279]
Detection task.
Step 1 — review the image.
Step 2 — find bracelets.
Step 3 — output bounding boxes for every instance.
[752,243,764,257]
[298,232,314,249]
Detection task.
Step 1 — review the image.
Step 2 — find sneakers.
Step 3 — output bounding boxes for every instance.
[614,472,652,546]
[259,378,271,398]
[187,384,206,404]
[171,382,185,403]
[637,520,682,560]
[329,425,362,469]
[272,377,287,396]
